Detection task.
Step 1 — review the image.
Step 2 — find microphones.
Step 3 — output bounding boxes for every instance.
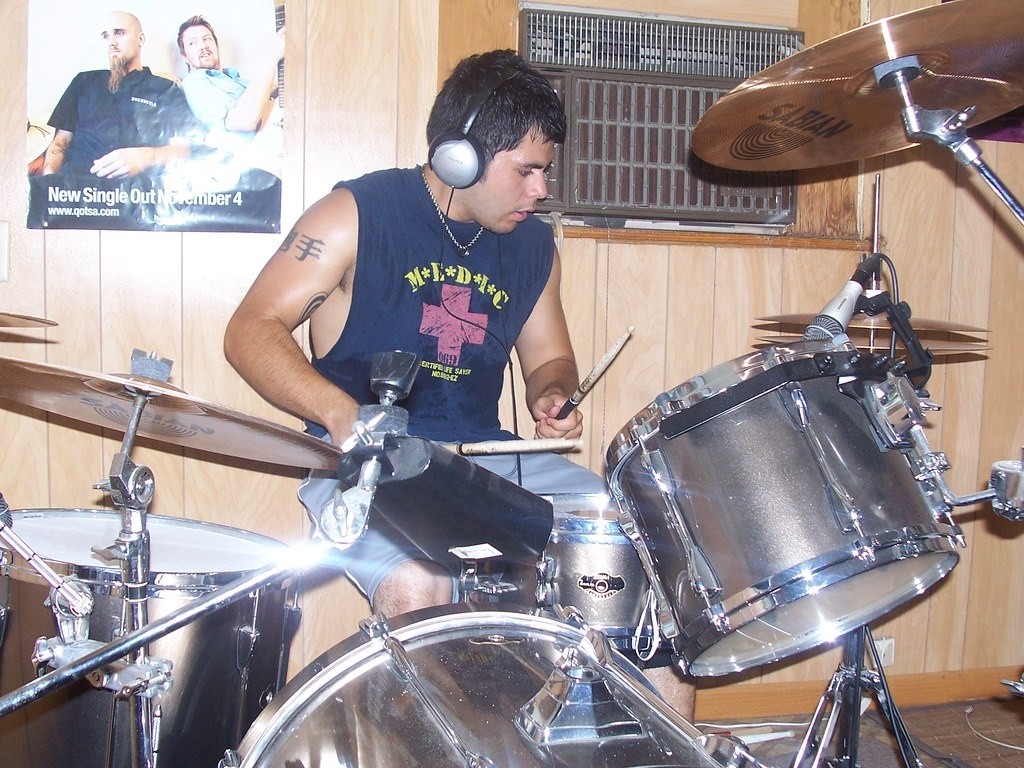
[803,253,879,339]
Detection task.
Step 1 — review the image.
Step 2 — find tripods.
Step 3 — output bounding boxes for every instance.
[793,626,924,768]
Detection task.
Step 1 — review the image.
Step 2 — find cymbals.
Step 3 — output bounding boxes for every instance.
[754,310,993,351]
[0,356,344,471]
[0,311,59,328]
[691,0,1024,172]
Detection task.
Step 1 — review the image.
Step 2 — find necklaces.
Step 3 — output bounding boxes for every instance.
[421,162,484,258]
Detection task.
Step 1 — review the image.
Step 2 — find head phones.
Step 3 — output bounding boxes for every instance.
[428,64,519,189]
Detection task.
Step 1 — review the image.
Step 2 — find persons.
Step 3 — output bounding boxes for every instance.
[27,12,204,230]
[222,51,749,752]
[176,16,285,183]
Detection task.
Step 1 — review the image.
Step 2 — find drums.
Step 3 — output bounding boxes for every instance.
[489,492,678,651]
[605,338,961,678]
[217,601,769,768]
[0,508,298,768]
